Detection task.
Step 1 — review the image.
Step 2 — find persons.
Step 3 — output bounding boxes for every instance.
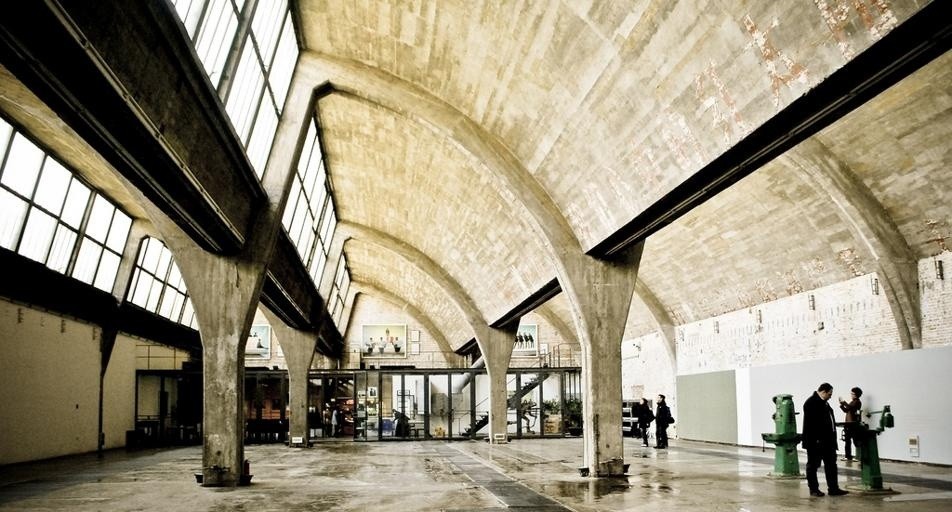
[839,387,864,460]
[330,409,338,438]
[638,394,669,448]
[803,381,849,497]
[366,327,403,356]
[392,409,409,437]
[256,338,268,350]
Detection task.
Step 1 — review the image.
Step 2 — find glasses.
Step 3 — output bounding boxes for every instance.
[851,393,856,396]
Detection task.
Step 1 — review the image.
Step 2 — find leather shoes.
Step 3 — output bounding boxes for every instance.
[828,489,846,496]
[810,491,825,497]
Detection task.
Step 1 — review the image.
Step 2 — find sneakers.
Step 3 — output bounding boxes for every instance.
[641,444,648,447]
[653,444,665,449]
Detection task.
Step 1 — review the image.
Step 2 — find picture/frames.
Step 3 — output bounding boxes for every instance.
[509,323,540,360]
[360,323,408,359]
[241,324,272,361]
